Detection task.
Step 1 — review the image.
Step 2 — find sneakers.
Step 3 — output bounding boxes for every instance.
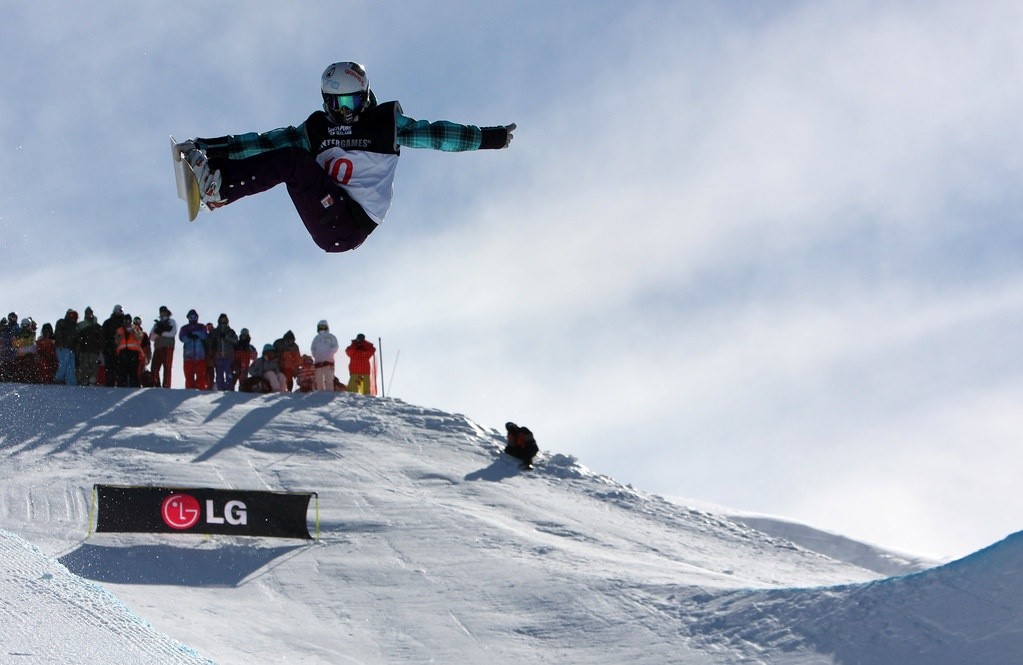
[184,150,229,212]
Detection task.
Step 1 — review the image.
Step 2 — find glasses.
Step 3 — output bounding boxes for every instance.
[324,92,366,111]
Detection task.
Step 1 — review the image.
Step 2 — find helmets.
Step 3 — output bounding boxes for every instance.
[322,62,372,125]
[8,312,19,321]
[21,318,32,327]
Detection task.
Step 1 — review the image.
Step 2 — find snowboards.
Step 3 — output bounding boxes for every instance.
[169,134,201,222]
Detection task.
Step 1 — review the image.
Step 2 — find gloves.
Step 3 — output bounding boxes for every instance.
[173,138,195,162]
[186,330,200,340]
[501,123,516,149]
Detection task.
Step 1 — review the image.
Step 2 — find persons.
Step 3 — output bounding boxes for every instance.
[0,312,56,384]
[179,310,207,389]
[312,320,338,391]
[204,323,216,390]
[149,306,177,388]
[55,309,78,385]
[273,331,299,393]
[208,314,239,391]
[296,355,315,391]
[346,334,376,394]
[236,328,257,386]
[505,422,538,468]
[76,307,101,385]
[102,304,151,388]
[171,61,517,252]
[249,344,287,394]
